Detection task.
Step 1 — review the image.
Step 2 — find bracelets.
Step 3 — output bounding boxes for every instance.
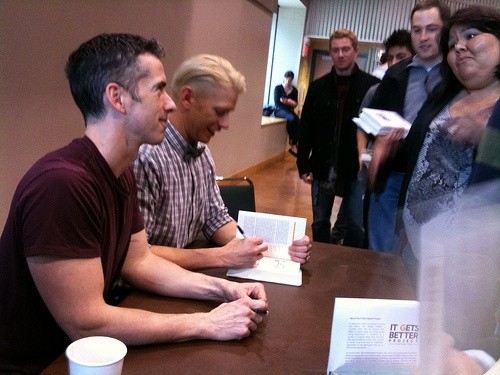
[284,99,288,104]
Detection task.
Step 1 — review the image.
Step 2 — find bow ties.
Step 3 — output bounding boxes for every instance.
[183,144,206,162]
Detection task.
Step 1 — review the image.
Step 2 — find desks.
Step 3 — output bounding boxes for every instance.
[38,242,418,375]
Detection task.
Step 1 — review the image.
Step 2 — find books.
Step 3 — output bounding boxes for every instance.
[226,210,308,286]
[352,107,412,140]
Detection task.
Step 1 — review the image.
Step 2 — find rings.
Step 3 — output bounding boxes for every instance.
[306,256,312,261]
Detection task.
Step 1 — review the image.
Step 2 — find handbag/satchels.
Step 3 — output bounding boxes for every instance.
[263,105,274,116]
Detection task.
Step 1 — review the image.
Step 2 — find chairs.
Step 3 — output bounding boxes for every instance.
[214,176,256,223]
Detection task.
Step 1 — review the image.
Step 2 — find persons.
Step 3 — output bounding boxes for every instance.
[361,0,451,255]
[129,52,313,269]
[273,70,299,157]
[342,29,415,248]
[0,33,269,375]
[367,6,500,303]
[371,53,389,80]
[434,98,500,375]
[297,29,382,245]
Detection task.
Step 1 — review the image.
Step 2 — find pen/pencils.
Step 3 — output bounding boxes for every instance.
[252,308,269,315]
[236,225,248,239]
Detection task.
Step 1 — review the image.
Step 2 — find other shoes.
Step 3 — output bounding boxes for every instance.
[289,148,298,156]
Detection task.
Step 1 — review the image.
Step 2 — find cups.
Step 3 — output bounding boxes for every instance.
[65,336,128,375]
